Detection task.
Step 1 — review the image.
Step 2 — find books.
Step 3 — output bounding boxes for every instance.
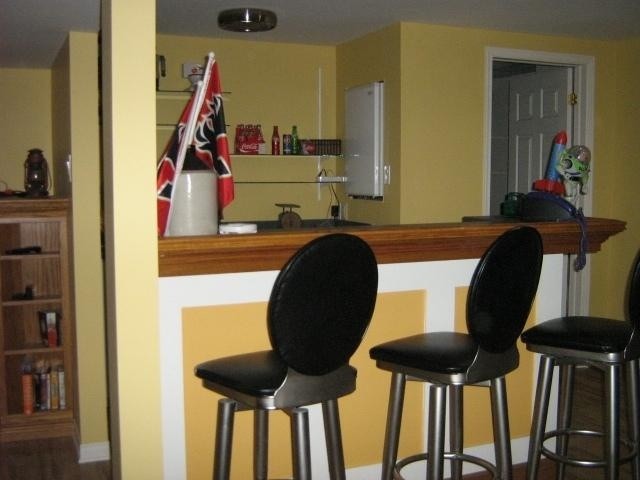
[39,311,61,347]
[22,363,66,415]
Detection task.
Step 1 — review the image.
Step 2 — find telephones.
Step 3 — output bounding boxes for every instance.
[519,192,576,222]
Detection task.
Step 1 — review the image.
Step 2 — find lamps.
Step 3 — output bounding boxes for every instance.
[218,8,278,33]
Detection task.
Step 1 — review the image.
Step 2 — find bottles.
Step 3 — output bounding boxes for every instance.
[271,125,280,154]
[234,122,266,155]
[291,126,298,154]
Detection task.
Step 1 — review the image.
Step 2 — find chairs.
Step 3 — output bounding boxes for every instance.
[520,247,639,480]
[370,224,545,480]
[193,234,379,479]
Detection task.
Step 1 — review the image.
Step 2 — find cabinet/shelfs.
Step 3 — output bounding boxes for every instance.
[0,193,72,442]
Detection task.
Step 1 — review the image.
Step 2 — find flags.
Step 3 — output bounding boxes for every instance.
[155,60,238,239]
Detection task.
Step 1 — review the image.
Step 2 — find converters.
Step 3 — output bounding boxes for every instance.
[332,206,338,216]
